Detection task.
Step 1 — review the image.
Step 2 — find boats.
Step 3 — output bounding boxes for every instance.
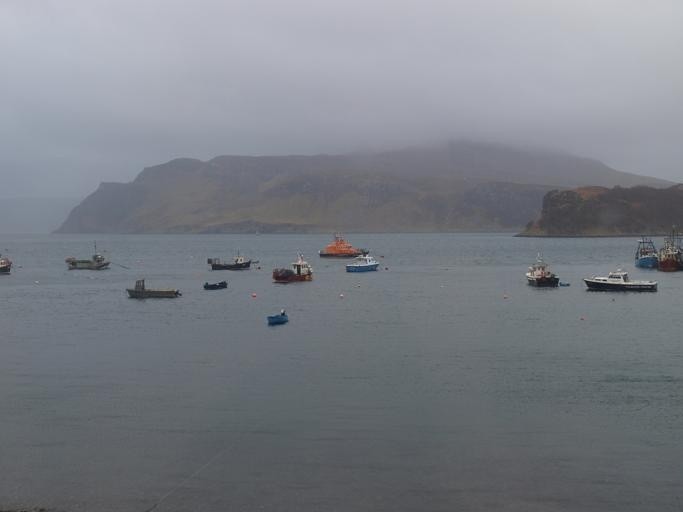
[265,308,289,327]
[124,277,179,299]
[204,247,252,273]
[343,250,379,274]
[269,251,315,284]
[631,234,659,269]
[0,253,14,274]
[201,280,229,291]
[523,251,560,288]
[317,231,370,258]
[655,223,682,271]
[581,267,659,292]
[63,240,110,270]
